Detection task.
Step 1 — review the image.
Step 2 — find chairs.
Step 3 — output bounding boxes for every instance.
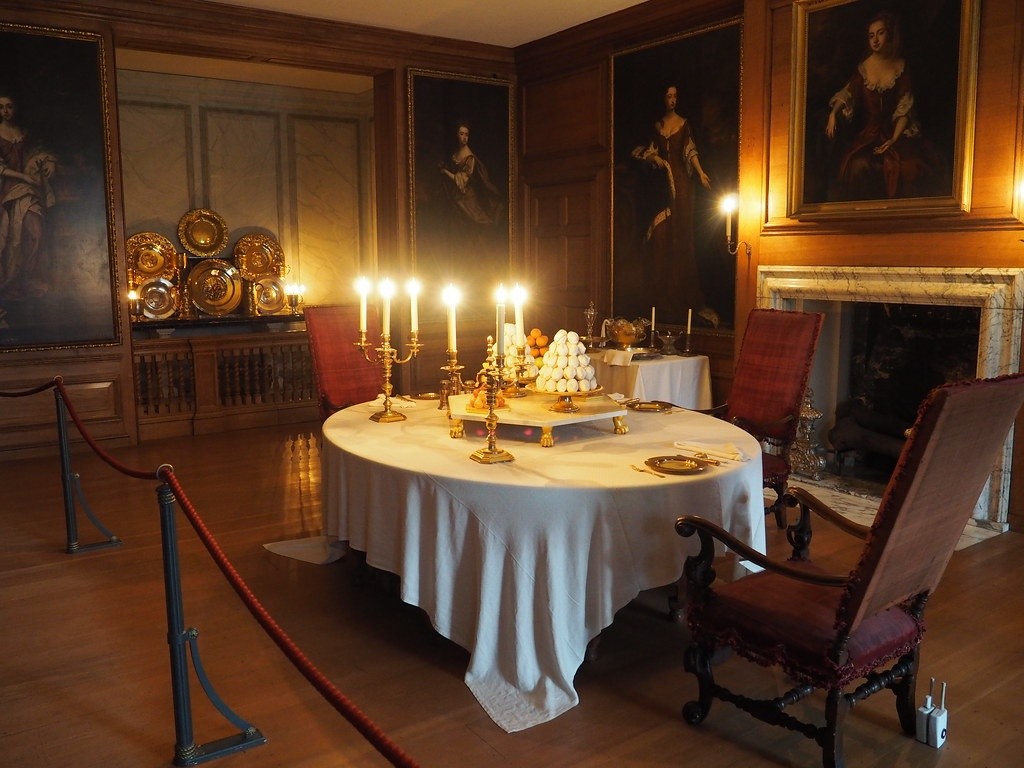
[677,371,1023,767]
[688,308,831,525]
[303,303,393,415]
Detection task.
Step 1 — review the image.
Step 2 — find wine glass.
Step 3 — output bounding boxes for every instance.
[288,295,299,319]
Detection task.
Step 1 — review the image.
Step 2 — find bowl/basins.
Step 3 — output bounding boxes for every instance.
[613,346,662,361]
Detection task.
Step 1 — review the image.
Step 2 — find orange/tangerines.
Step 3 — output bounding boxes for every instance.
[526,329,549,358]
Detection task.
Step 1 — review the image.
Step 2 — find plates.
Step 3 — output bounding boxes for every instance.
[233,232,286,281]
[244,277,289,314]
[125,232,176,286]
[186,258,244,316]
[177,208,230,256]
[124,274,180,320]
[645,454,708,475]
[626,400,672,412]
[408,392,443,401]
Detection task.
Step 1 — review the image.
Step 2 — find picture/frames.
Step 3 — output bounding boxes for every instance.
[1,14,124,354]
[789,1,977,220]
[410,64,520,308]
[608,17,742,327]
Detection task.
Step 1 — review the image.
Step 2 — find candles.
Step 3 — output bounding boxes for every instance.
[376,278,396,334]
[511,283,529,345]
[438,284,464,349]
[405,277,424,331]
[354,275,372,331]
[652,306,654,331]
[688,308,692,334]
[493,282,509,354]
[721,195,736,239]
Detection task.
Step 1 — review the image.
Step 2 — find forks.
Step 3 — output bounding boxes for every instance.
[629,464,667,479]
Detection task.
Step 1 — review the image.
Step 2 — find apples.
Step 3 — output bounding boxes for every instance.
[535,357,544,369]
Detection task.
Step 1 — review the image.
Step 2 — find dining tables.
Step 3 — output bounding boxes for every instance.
[322,395,770,736]
[585,350,711,408]
